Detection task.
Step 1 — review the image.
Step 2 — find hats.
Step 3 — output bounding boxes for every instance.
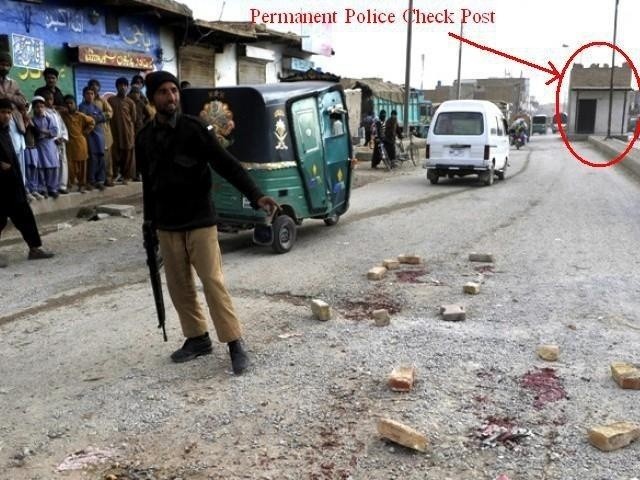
[31,94,47,103]
[145,70,179,103]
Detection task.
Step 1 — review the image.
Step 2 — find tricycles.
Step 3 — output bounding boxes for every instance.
[182,78,355,253]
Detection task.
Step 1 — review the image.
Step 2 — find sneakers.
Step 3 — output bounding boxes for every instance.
[42,177,129,199]
[25,193,37,201]
[32,192,45,200]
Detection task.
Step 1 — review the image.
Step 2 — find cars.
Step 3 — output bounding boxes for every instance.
[627,116,639,134]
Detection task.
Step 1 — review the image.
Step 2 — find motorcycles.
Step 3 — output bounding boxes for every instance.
[509,125,528,151]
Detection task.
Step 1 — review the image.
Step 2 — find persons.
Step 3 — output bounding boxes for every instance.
[508,114,527,148]
[134,71,284,378]
[359,107,403,170]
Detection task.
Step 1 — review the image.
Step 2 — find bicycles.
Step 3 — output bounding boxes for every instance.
[377,139,393,171]
[394,132,418,169]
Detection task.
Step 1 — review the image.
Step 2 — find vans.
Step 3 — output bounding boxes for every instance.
[532,114,549,135]
[553,111,568,133]
[418,98,511,186]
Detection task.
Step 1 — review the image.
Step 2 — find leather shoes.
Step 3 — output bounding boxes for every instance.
[28,247,55,260]
[170,332,212,363]
[228,336,250,375]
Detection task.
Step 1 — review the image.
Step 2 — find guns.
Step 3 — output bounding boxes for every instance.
[141,219,169,344]
